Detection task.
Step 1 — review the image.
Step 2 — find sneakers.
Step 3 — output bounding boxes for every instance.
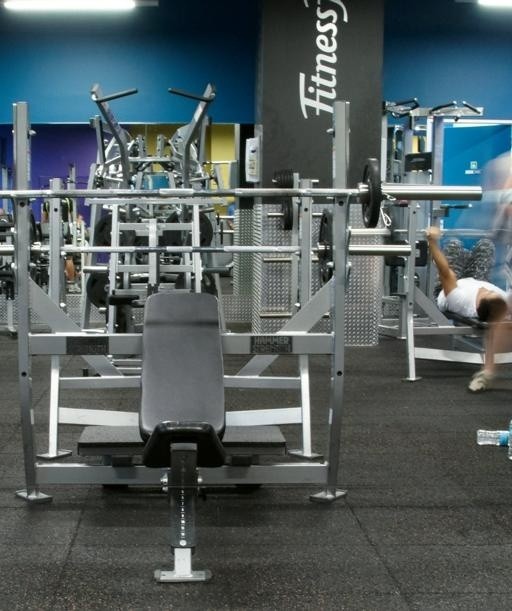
[468,372,499,391]
[67,284,82,293]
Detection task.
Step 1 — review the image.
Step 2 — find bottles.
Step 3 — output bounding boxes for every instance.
[477,429,510,446]
[507,417,512,460]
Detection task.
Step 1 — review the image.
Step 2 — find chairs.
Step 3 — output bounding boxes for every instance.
[135,292,228,584]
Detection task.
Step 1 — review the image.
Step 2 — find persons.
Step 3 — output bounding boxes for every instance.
[423,226,511,326]
[42,185,83,294]
[471,154,512,390]
